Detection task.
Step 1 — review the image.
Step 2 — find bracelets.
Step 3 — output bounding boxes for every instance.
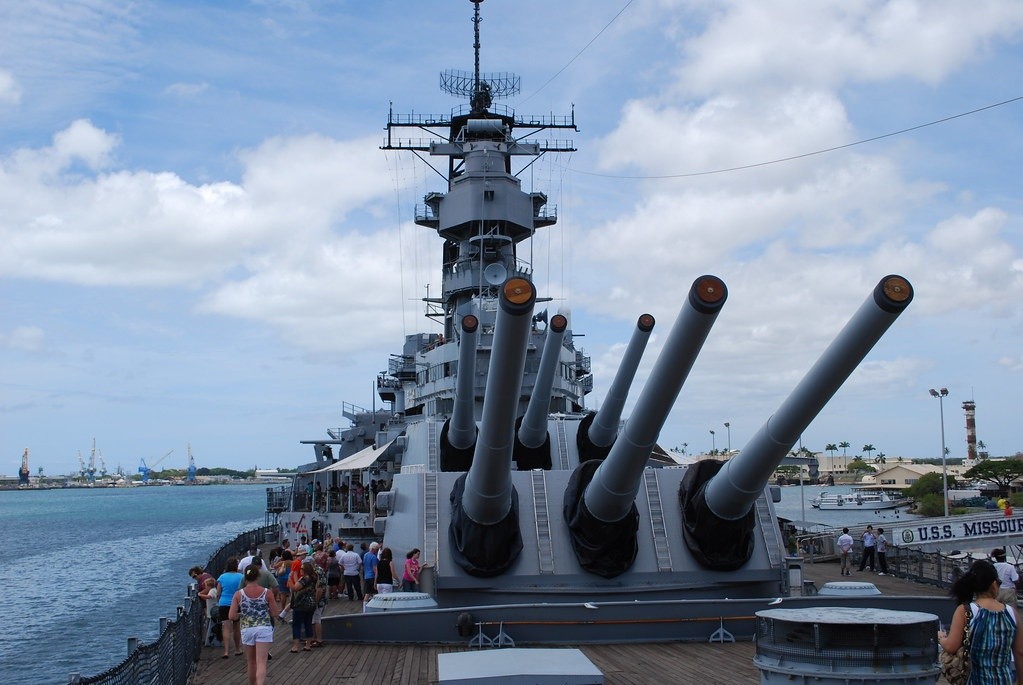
[938,635,947,642]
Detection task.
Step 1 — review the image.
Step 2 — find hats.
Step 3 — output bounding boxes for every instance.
[294,549,308,556]
[311,539,317,544]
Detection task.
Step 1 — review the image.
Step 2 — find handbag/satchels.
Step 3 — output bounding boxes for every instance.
[939,602,973,685]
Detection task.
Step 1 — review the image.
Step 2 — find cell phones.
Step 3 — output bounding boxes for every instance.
[866,530,870,532]
[331,539,333,543]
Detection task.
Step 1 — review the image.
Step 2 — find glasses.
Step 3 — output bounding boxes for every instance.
[192,573,199,578]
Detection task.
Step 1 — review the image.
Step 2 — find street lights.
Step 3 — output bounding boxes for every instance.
[929,388,949,517]
[724,422,731,459]
[709,430,715,458]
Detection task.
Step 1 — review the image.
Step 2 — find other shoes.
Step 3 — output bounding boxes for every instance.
[338,593,348,597]
[221,654,228,658]
[276,616,293,624]
[290,648,311,652]
[878,572,886,576]
[876,571,881,575]
[268,652,272,660]
[235,651,244,655]
[846,571,850,575]
[841,570,844,574]
[869,569,873,572]
[857,569,863,571]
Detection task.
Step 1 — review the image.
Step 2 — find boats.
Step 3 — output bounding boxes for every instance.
[809,490,900,510]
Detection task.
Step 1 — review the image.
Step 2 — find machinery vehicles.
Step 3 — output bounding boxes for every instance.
[951,494,973,507]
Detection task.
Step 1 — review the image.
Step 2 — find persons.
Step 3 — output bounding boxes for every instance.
[377,377,400,388]
[787,529,799,557]
[437,334,443,347]
[876,528,888,575]
[837,527,853,575]
[189,533,428,685]
[856,525,876,571]
[296,478,393,513]
[937,548,1023,685]
[985,494,1013,518]
